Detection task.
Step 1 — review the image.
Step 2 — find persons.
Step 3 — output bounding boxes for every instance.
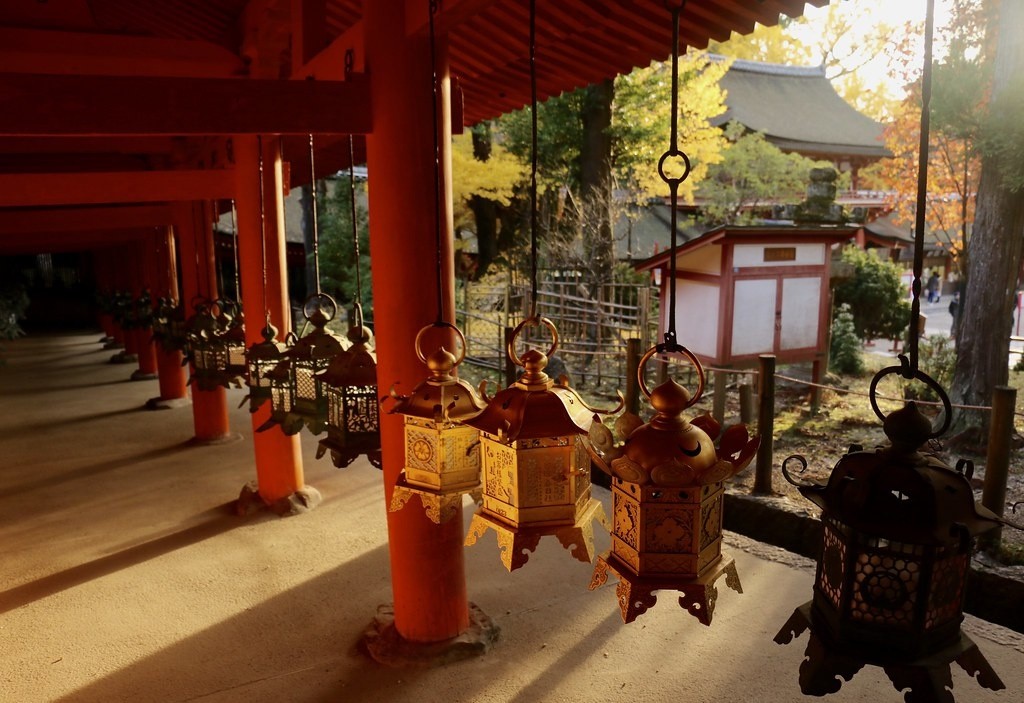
[927,271,941,303]
[948,291,959,335]
